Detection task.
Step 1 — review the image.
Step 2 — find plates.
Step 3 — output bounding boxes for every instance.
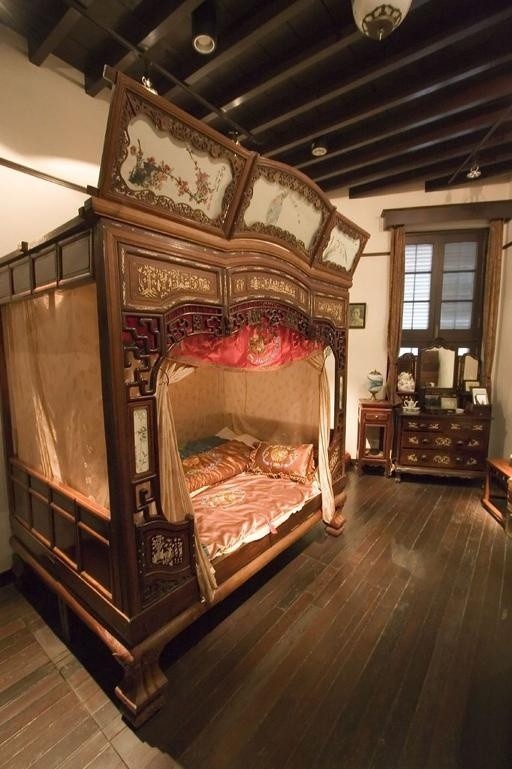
[402,406,421,410]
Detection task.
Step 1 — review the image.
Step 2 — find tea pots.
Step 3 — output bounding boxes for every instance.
[403,398,419,408]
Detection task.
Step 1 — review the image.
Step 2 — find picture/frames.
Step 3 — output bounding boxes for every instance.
[470,386,488,406]
[345,301,367,330]
[464,379,480,394]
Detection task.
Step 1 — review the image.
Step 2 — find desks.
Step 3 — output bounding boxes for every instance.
[355,398,396,480]
[480,457,512,534]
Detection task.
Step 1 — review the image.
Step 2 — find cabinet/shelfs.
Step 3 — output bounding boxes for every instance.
[398,412,494,487]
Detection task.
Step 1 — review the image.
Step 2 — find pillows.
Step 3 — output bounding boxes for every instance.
[246,432,320,487]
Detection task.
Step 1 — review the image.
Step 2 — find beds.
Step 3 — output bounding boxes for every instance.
[0,64,374,731]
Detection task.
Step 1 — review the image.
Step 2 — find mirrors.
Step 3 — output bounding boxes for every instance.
[459,353,482,390]
[416,337,458,394]
[395,351,418,397]
